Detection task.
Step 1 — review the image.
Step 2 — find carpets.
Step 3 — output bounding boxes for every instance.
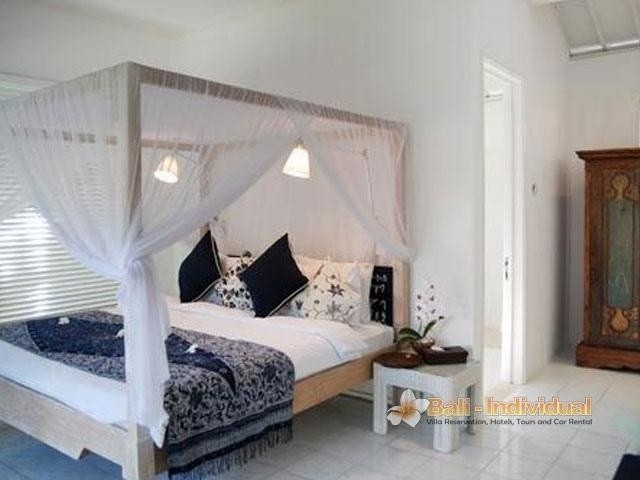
[612,452,640,480]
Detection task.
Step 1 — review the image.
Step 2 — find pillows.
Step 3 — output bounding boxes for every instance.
[177,229,375,331]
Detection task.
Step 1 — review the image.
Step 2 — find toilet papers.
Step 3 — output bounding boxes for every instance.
[423,344,470,365]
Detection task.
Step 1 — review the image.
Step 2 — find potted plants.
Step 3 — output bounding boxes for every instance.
[393,315,445,353]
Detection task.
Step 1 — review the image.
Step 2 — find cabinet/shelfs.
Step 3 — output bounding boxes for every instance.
[574,147,640,372]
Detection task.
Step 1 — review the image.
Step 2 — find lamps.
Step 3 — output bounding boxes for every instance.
[281,136,311,181]
[154,142,182,184]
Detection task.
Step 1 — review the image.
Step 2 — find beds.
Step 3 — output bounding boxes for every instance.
[0,61,410,480]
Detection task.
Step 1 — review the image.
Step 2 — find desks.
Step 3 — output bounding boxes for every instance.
[371,352,483,453]
